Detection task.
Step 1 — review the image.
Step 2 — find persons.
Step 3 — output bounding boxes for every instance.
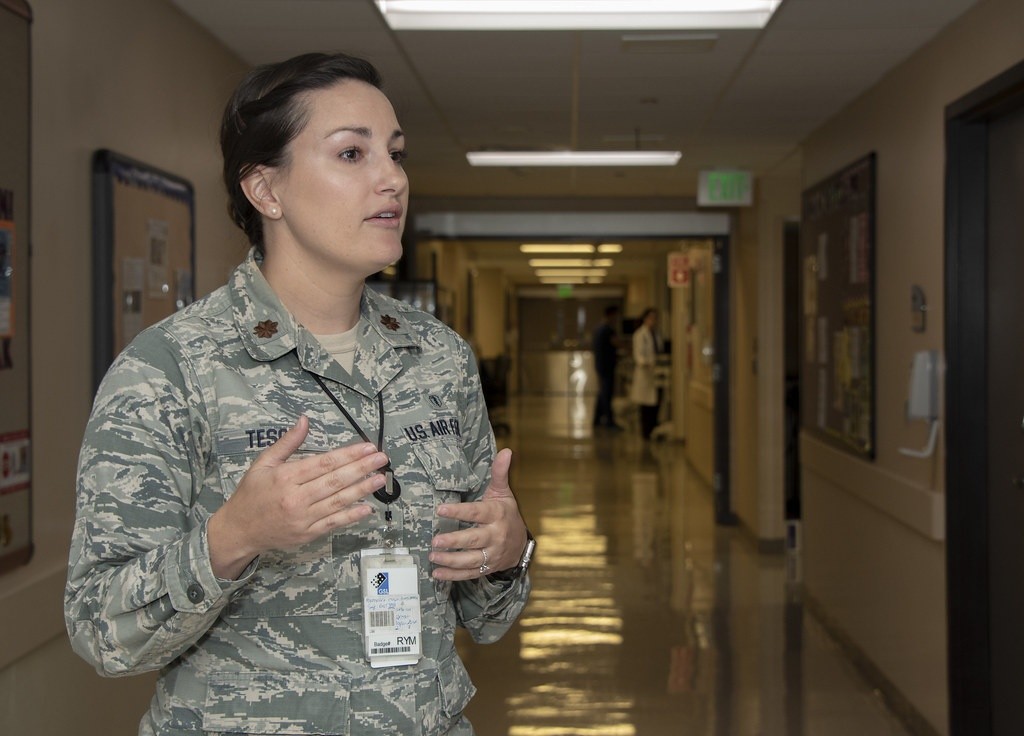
[593,305,628,431]
[64,50,535,736]
[632,308,667,444]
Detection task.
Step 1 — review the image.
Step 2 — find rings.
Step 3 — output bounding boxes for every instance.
[479,547,490,573]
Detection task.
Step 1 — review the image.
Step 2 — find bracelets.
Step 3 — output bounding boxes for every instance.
[486,529,536,582]
